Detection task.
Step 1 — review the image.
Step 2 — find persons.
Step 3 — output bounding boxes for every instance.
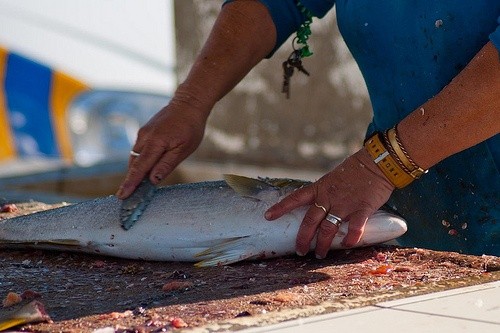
[113,0,500,257]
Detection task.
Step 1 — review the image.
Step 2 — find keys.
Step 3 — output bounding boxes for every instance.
[281,49,310,98]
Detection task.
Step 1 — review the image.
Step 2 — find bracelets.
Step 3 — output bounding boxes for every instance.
[364,132,414,188]
[383,122,429,179]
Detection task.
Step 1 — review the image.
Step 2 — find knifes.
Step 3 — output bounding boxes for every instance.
[119,180,155,230]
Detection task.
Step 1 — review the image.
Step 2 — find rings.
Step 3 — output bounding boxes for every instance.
[130,149,139,157]
[326,215,342,227]
[313,201,328,214]
[328,212,342,222]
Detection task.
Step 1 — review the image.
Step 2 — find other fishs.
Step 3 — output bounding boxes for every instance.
[0,173,408,269]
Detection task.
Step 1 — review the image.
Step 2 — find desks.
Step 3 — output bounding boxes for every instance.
[0,196,500,331]
